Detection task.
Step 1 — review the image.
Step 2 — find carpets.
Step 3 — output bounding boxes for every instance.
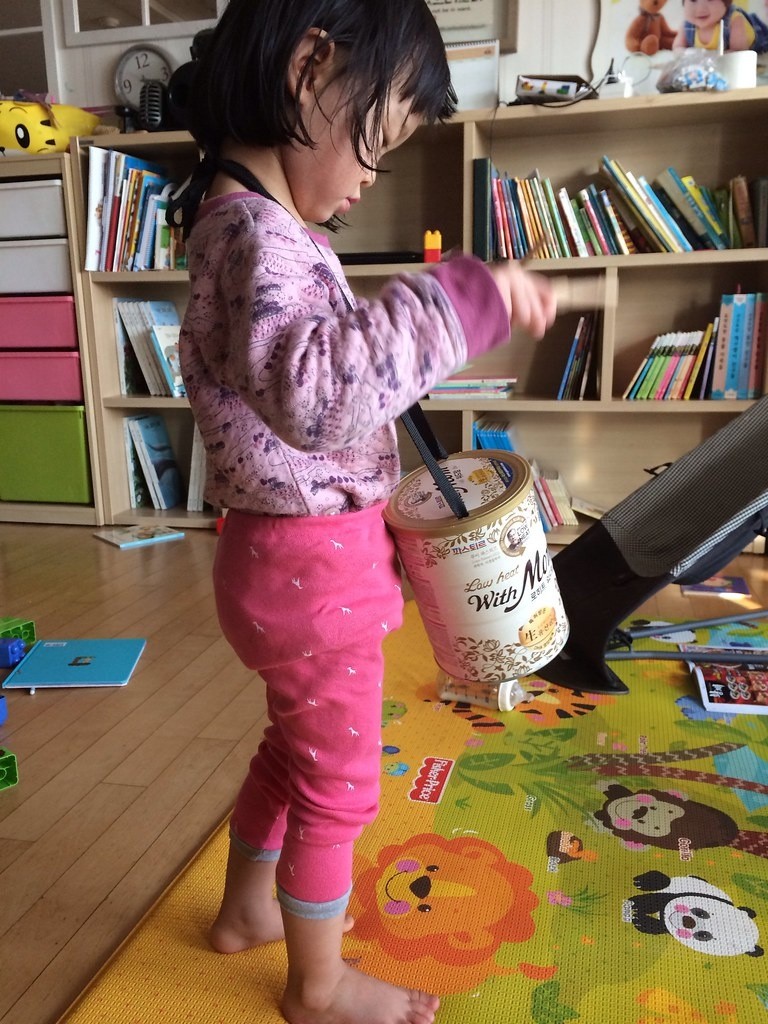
[54,602,768,1024]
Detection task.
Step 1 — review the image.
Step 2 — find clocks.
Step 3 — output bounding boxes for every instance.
[113,43,180,113]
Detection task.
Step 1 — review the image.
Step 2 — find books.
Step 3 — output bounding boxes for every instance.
[123,414,208,511]
[427,376,518,403]
[622,291,768,399]
[92,523,185,549]
[111,297,186,397]
[678,643,768,714]
[474,414,608,533]
[681,575,752,598]
[84,146,181,272]
[556,316,599,400]
[473,154,767,263]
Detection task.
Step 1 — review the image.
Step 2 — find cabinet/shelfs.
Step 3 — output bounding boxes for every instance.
[0,87,768,560]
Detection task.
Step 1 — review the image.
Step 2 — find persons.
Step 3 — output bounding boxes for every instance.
[177,0,556,1023]
[672,0,768,54]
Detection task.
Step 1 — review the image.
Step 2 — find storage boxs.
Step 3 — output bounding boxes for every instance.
[0,238,72,294]
[0,296,80,349]
[0,179,68,240]
[0,351,84,401]
[0,403,91,503]
[516,73,599,100]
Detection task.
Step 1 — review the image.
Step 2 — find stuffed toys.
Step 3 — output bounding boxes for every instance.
[625,0,678,55]
